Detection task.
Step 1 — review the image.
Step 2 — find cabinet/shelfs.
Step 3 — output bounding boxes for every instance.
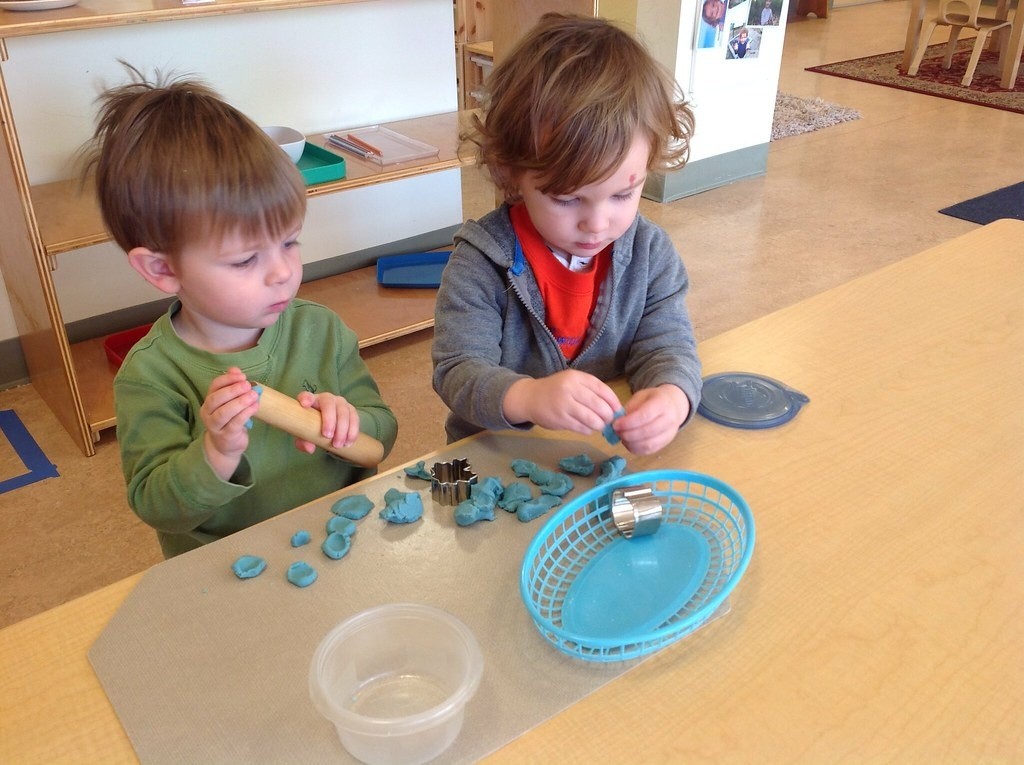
[0,0,597,459]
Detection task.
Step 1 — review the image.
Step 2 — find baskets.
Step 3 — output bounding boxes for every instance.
[519,469,755,661]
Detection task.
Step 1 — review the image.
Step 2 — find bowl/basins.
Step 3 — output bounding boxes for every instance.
[306,603,485,765]
[262,126,306,168]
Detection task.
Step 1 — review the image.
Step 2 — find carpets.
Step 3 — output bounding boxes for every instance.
[938,182,1024,225]
[770,91,862,141]
[805,35,1024,116]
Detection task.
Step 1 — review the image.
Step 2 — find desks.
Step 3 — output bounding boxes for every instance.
[903,0,1024,90]
[1,219,1024,765]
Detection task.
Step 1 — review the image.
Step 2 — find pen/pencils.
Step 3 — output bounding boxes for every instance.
[328,133,383,160]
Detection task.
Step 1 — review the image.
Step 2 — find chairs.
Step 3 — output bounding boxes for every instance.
[907,0,1012,85]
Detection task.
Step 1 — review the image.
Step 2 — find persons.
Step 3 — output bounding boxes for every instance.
[734,29,751,59]
[761,0,773,26]
[73,60,399,562]
[702,0,728,31]
[432,12,704,454]
